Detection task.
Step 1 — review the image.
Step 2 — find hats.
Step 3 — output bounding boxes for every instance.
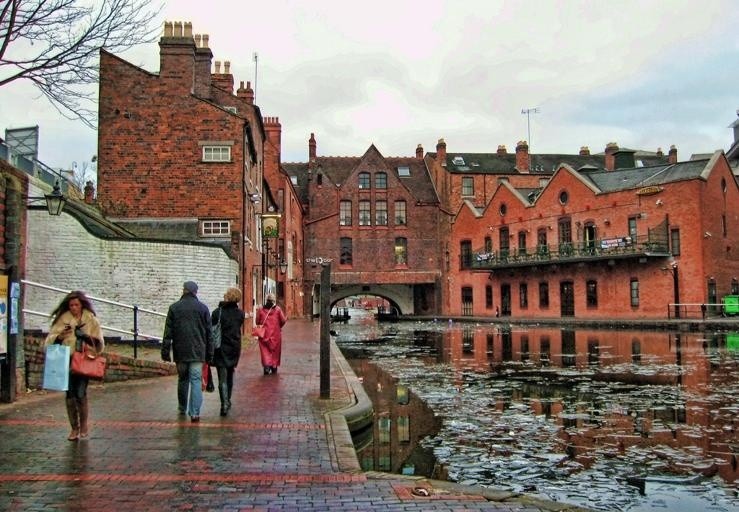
[183,280,199,294]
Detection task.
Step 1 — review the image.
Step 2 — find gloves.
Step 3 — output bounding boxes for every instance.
[160,348,172,362]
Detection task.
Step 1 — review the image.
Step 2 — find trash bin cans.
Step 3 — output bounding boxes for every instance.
[378,306,383,313]
[723,296,738,314]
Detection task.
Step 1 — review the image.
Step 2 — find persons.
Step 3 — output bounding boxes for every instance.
[211,288,245,415]
[43,291,105,441]
[162,282,215,422]
[256,294,287,375]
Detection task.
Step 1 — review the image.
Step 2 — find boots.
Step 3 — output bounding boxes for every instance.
[217,383,234,416]
[76,397,89,437]
[64,398,79,440]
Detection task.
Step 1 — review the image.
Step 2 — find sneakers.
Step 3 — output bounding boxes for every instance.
[179,409,187,415]
[190,414,200,422]
[264,368,278,375]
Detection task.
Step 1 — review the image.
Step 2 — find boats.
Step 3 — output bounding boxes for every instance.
[331,306,352,322]
[374,306,397,320]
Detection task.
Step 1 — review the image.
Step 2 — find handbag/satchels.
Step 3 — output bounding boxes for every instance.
[42,344,70,391]
[70,337,107,380]
[252,328,265,338]
[207,364,215,394]
[202,362,209,391]
[211,320,223,350]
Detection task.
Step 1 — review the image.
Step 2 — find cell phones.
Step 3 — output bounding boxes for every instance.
[65,325,71,329]
